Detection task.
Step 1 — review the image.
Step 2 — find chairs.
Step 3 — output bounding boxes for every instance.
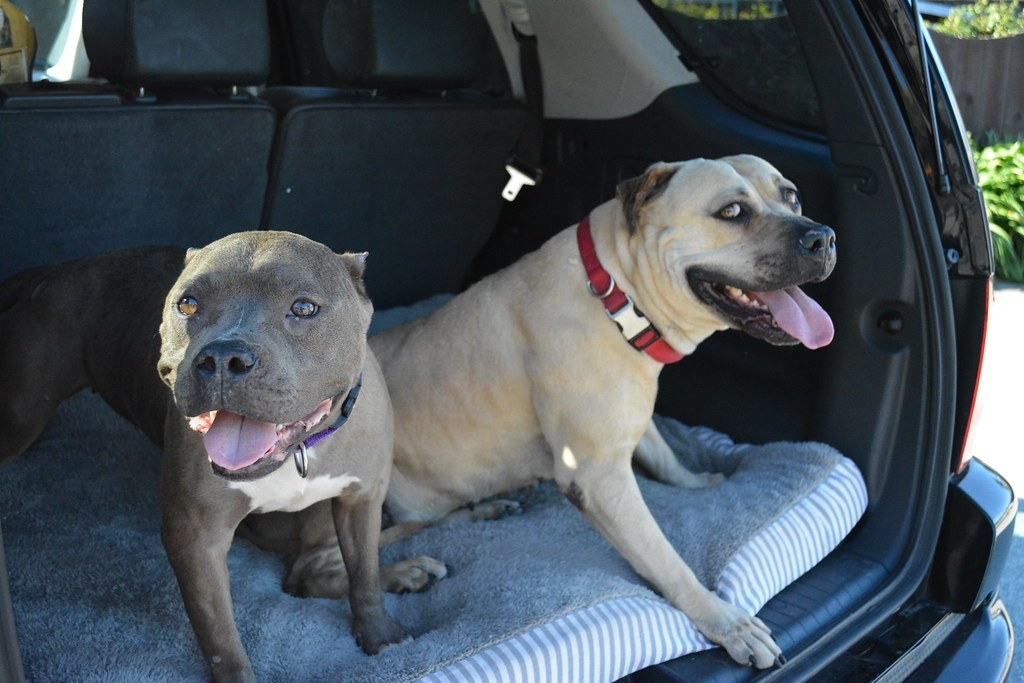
[0,0,531,315]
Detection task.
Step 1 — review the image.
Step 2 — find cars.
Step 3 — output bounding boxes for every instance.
[0,0,1024,683]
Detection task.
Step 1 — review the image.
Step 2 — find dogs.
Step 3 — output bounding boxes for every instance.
[0,149,840,681]
